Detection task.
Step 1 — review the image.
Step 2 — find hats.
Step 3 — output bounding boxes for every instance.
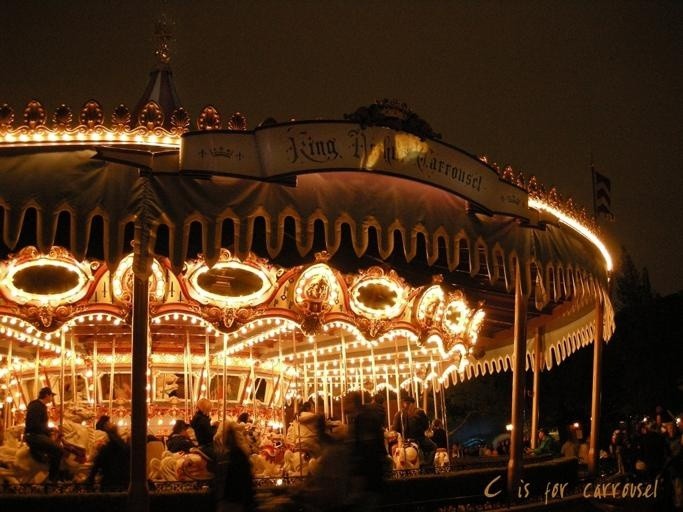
[168,420,190,437]
[402,396,415,404]
[96,415,110,431]
[39,387,58,397]
[197,398,212,418]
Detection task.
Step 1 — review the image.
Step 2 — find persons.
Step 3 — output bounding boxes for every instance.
[2,403,452,501]
[342,391,396,512]
[189,398,223,472]
[0,402,451,501]
[203,420,264,511]
[390,396,437,475]
[453,414,682,512]
[367,394,388,427]
[22,386,69,480]
